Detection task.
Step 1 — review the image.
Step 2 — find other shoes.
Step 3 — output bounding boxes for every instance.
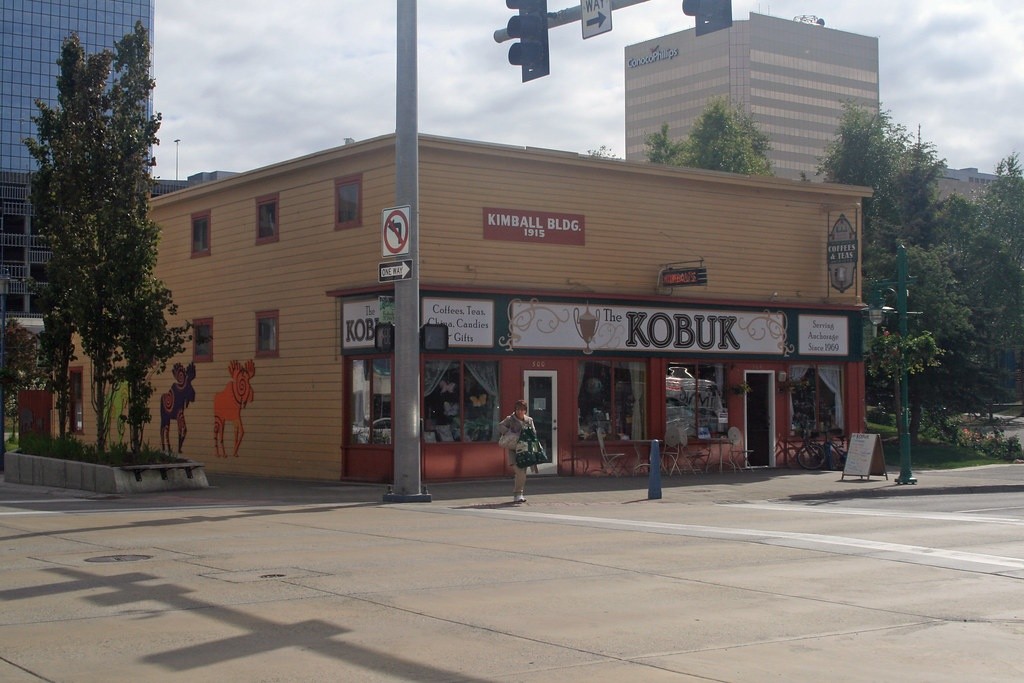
[513,496,526,502]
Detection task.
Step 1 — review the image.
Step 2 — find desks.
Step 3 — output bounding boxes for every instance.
[629,439,667,477]
[689,441,733,473]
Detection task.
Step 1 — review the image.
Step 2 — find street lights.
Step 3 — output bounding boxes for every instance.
[867,243,919,485]
[174,139,180,180]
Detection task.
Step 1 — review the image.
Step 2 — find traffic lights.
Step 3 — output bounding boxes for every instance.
[682,0,734,37]
[506,0,551,85]
[420,323,450,353]
[374,323,395,353]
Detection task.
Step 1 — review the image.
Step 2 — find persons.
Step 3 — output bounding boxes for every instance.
[496,399,537,502]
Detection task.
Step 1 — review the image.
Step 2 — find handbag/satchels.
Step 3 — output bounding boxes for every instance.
[498,428,520,450]
[516,426,548,468]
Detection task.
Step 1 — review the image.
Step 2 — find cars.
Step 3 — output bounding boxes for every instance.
[372,417,392,445]
[664,366,716,408]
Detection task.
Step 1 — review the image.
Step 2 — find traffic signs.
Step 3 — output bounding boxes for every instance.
[581,0,613,40]
[378,258,415,282]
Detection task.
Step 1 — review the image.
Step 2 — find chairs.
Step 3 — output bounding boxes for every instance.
[596,427,754,478]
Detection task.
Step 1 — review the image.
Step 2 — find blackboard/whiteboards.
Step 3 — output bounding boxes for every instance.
[843,432,886,476]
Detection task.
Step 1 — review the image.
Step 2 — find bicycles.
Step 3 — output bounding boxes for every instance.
[796,428,850,471]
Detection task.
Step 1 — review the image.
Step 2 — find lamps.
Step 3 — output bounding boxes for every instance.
[422,322,449,351]
[374,322,395,351]
